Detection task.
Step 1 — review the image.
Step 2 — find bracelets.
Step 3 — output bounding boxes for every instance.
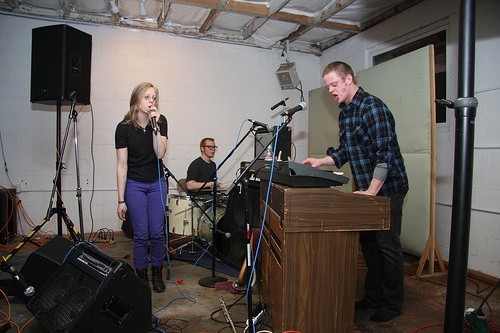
[119,200,125,204]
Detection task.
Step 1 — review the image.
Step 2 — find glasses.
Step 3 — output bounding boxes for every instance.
[203,146,218,150]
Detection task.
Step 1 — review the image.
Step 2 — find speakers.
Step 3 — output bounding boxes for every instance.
[11,235,153,333]
[254,125,291,161]
[30,24,92,105]
[276,62,300,90]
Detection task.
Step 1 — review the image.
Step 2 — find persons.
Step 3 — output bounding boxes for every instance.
[303,61,409,322]
[115,82,169,292]
[186,138,227,193]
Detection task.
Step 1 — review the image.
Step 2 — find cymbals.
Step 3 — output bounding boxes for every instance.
[177,178,187,196]
[190,189,227,195]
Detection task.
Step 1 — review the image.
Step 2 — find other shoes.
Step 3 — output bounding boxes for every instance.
[370,309,395,323]
[355,298,374,309]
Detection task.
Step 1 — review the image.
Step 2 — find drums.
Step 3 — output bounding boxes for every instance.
[167,195,209,240]
[198,204,235,262]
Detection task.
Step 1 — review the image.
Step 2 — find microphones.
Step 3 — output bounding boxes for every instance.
[6,260,35,296]
[281,102,308,117]
[148,110,158,135]
[271,98,289,110]
[209,227,231,238]
[247,118,274,132]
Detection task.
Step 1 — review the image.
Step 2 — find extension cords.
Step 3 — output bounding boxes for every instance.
[93,238,116,243]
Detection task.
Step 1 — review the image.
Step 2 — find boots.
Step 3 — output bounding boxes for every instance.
[136,267,149,287]
[151,265,166,292]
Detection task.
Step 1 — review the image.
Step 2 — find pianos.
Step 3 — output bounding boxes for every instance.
[245,157,350,189]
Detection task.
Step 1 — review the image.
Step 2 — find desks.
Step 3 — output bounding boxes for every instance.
[258,179,390,333]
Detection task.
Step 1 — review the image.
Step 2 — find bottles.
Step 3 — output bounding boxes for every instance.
[264,145,273,168]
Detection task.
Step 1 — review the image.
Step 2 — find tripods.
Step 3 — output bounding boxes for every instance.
[4,105,83,261]
[169,199,221,264]
